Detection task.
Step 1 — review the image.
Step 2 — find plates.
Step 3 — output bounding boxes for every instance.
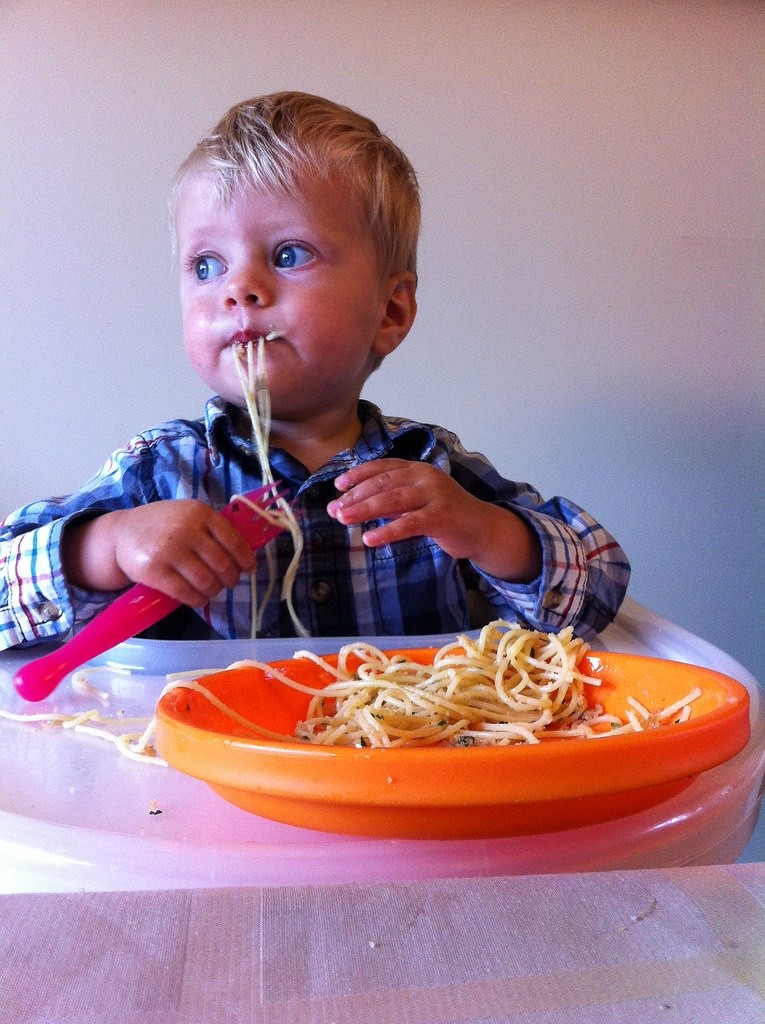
[157,647,752,841]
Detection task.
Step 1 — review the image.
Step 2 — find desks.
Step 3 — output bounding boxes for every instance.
[1,596,764,886]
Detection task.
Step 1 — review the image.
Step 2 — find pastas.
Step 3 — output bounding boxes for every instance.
[0,330,704,750]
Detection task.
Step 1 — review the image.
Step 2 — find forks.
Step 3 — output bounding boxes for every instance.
[13,479,308,703]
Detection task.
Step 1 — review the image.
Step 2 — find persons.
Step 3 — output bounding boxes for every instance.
[0,90,630,656]
